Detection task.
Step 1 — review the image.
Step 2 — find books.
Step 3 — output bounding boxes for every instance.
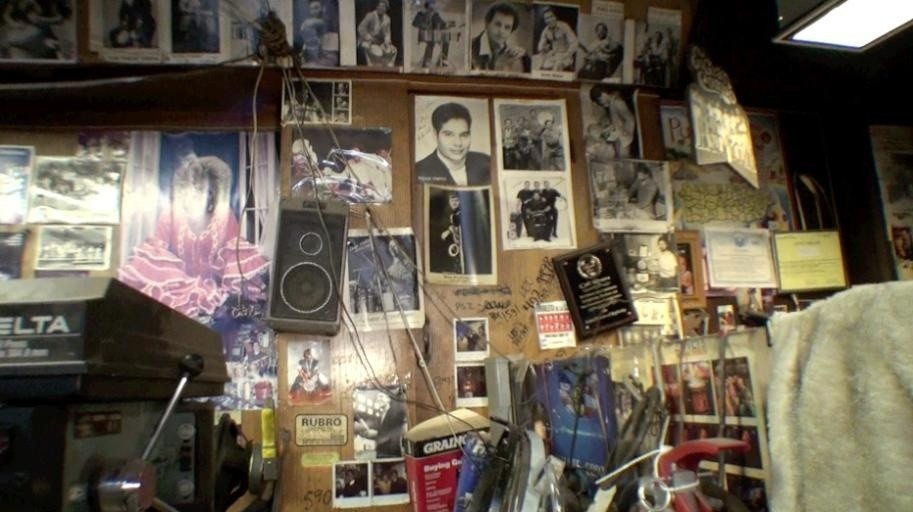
[403,408,491,512]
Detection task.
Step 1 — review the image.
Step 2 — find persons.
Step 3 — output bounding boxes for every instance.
[624,165,658,220]
[537,8,577,71]
[470,3,531,73]
[109,0,155,48]
[343,469,360,496]
[290,348,327,396]
[585,86,636,157]
[501,109,565,173]
[516,181,567,237]
[356,0,398,67]
[679,255,693,293]
[634,24,680,89]
[433,194,461,275]
[414,103,490,187]
[374,468,407,495]
[724,311,733,326]
[299,1,339,67]
[354,393,404,459]
[386,237,415,310]
[473,325,486,350]
[657,238,678,293]
[578,23,617,79]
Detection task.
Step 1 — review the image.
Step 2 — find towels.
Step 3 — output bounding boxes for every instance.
[766,280,913,512]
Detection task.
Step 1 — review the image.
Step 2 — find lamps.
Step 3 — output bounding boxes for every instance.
[771,0,913,53]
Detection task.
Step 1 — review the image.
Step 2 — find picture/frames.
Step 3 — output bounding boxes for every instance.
[675,230,706,309]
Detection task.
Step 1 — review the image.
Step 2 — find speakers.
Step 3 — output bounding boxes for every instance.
[264,196,350,337]
